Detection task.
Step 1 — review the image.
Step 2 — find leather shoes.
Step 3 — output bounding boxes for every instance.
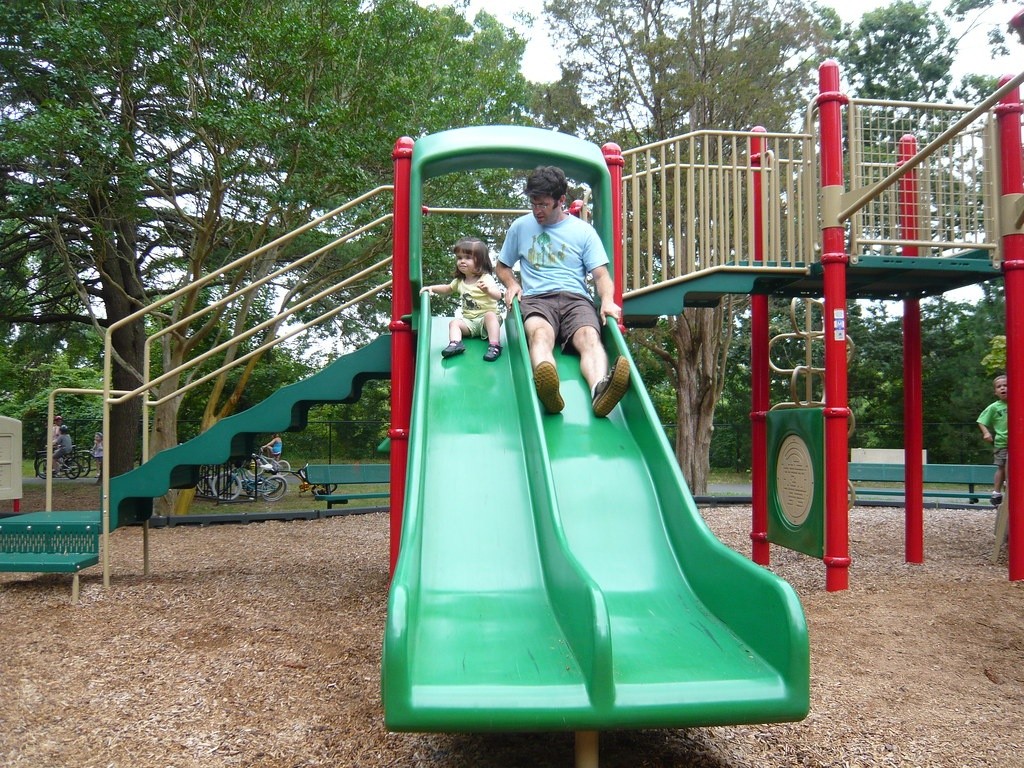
[442,340,464,357]
[483,344,503,362]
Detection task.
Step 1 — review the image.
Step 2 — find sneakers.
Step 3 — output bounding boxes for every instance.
[533,361,565,415]
[591,355,630,418]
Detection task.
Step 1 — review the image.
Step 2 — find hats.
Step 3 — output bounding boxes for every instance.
[95,431,103,440]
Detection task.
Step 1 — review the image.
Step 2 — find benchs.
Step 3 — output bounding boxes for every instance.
[847,462,1006,501]
[303,462,390,508]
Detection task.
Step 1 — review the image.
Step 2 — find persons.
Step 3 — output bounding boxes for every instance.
[52,415,103,477]
[418,236,503,362]
[495,166,630,418]
[261,433,282,462]
[977,375,1007,506]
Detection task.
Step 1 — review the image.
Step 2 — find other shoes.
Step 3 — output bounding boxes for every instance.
[94,473,100,477]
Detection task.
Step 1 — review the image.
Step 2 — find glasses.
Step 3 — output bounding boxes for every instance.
[527,203,556,210]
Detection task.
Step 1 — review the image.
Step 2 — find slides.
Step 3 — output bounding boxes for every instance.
[379,290,811,733]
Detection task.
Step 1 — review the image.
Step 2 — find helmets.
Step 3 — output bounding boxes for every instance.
[53,415,62,422]
[61,425,67,428]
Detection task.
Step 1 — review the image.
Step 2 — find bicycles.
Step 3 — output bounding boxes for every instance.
[34,442,92,480]
[196,445,338,503]
